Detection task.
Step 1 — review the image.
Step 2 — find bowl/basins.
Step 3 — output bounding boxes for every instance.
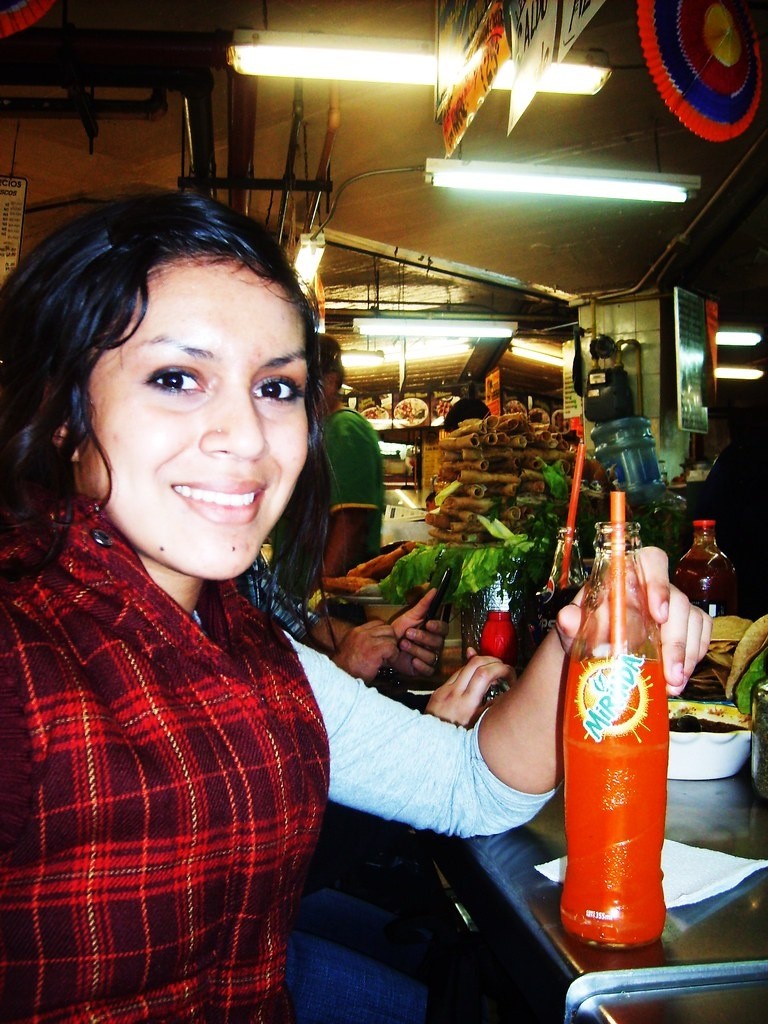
[665,702,754,782]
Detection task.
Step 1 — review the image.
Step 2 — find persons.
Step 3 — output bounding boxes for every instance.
[0,183,714,1024]
[237,332,518,978]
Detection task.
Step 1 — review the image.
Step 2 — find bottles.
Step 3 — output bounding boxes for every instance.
[673,519,739,621]
[537,526,588,644]
[589,415,667,510]
[481,611,516,667]
[657,460,670,486]
[466,648,511,709]
[560,522,670,950]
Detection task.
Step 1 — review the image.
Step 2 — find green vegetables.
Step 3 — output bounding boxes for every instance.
[375,455,576,606]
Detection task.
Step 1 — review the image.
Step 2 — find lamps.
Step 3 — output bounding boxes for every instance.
[226,30,613,97]
[424,156,702,205]
[353,317,519,339]
[292,234,327,295]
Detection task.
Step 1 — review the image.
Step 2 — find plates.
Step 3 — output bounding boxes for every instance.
[360,406,389,420]
[432,394,461,417]
[342,594,383,603]
[394,398,430,428]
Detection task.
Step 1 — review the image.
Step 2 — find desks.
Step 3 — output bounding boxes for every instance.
[464,777,768,1024]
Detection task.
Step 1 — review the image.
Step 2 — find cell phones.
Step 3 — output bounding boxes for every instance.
[419,567,452,631]
